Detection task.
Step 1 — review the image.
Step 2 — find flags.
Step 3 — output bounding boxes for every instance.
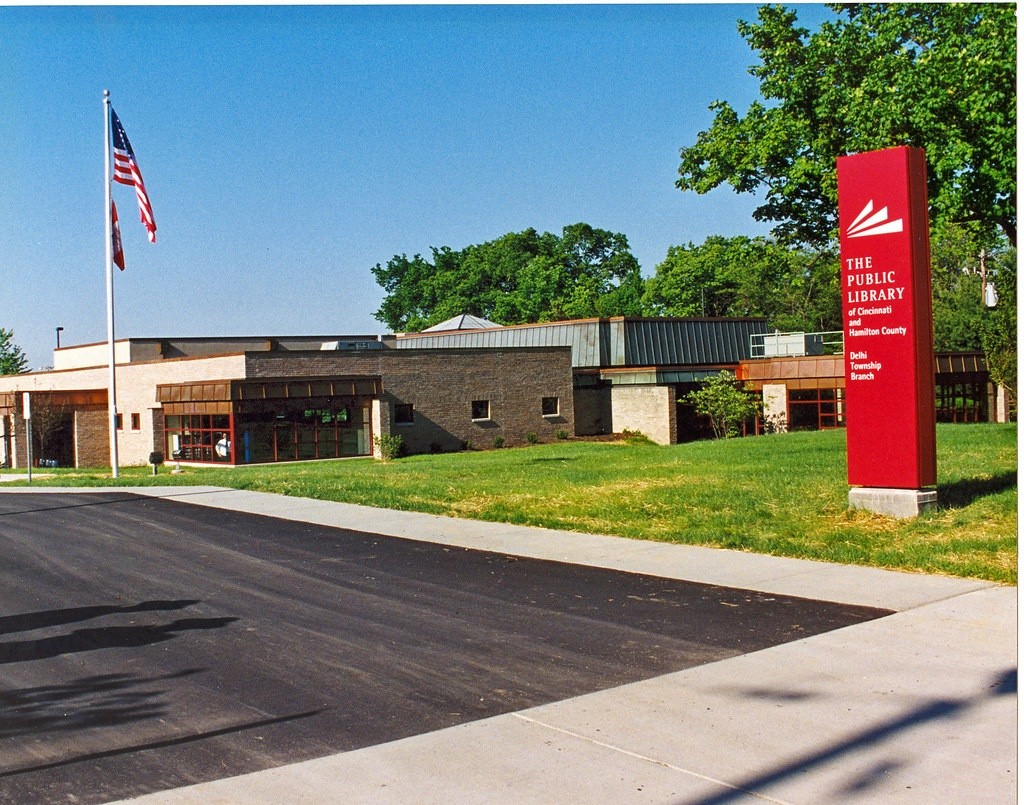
[111,199,125,271]
[111,103,157,242]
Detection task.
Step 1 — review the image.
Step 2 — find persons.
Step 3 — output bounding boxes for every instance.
[215,433,231,462]
